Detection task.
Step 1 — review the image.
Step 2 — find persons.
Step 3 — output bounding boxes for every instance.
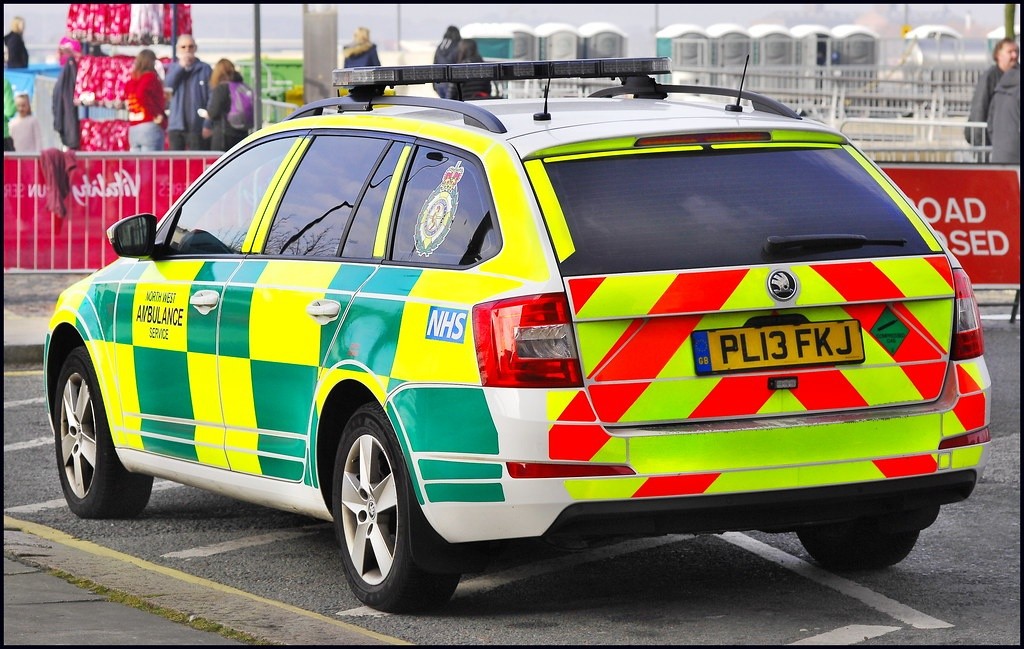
[3,16,30,68]
[430,26,493,103]
[123,31,250,153]
[8,93,42,153]
[965,35,1020,164]
[343,24,388,96]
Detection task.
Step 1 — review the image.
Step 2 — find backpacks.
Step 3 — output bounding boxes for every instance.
[220,80,253,131]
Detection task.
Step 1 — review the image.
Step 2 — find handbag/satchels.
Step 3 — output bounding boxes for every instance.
[146,94,168,129]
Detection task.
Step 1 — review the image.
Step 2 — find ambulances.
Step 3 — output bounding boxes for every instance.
[41,56,994,615]
